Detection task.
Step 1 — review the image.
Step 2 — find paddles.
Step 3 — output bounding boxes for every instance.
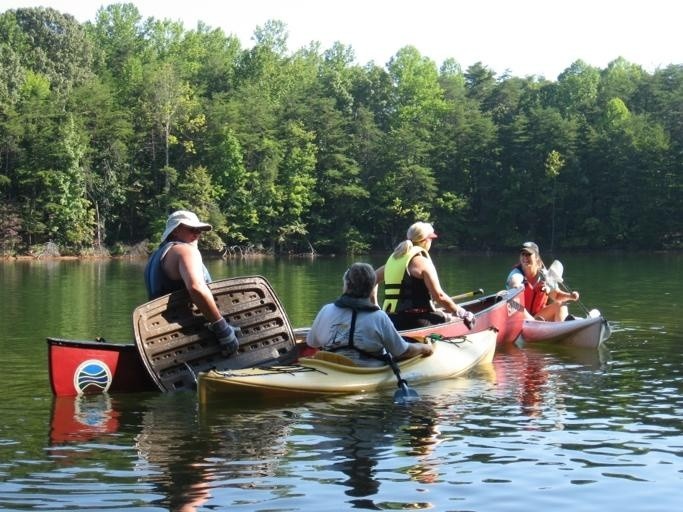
[385,352,418,400]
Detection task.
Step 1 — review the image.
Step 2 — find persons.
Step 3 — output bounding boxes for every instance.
[369,221,477,331]
[304,261,433,364]
[505,240,580,322]
[143,210,241,355]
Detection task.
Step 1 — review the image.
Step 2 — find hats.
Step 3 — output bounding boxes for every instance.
[412,231,438,245]
[519,240,540,255]
[159,209,212,243]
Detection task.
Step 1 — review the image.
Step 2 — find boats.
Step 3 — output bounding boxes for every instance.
[45,284,526,399]
[196,321,500,409]
[520,307,614,351]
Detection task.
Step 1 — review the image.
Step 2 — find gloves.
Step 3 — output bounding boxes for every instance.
[456,307,477,330]
[207,316,242,358]
